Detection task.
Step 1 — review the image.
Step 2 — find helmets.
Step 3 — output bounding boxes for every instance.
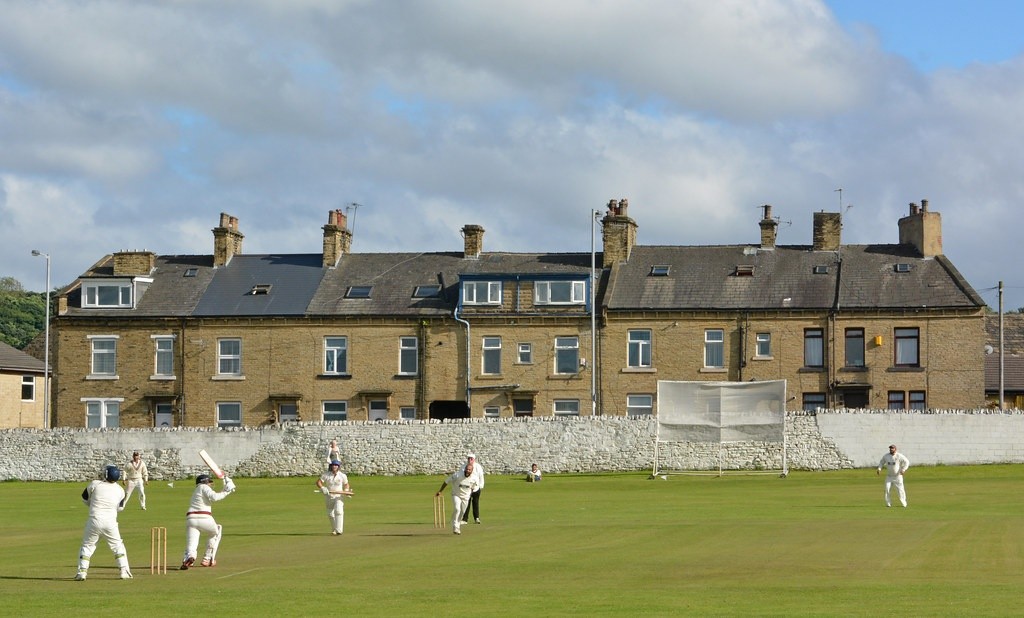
[103,465,120,483]
[196,475,213,484]
[331,460,340,467]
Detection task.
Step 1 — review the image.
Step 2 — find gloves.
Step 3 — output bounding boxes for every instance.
[225,481,236,491]
[223,477,232,486]
[344,489,353,498]
[320,486,330,495]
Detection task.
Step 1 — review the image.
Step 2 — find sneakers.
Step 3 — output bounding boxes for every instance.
[74,572,87,580]
[180,554,196,570]
[120,570,133,579]
[201,559,217,566]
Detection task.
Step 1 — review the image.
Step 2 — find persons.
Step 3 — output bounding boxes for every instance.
[990,401,999,410]
[876,444,910,508]
[73,465,134,581]
[315,459,353,536]
[180,474,237,571]
[325,440,341,472]
[460,453,484,524]
[118,452,149,510]
[527,463,542,482]
[435,464,479,536]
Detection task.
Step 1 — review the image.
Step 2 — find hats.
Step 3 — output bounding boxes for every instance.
[888,445,896,448]
[467,454,475,459]
[133,452,139,456]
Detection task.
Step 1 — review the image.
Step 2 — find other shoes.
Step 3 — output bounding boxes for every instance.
[336,530,343,534]
[453,518,480,535]
[142,507,146,510]
[332,530,336,534]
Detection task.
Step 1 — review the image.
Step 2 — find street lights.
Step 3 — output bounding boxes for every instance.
[31,248,54,428]
[591,207,604,414]
